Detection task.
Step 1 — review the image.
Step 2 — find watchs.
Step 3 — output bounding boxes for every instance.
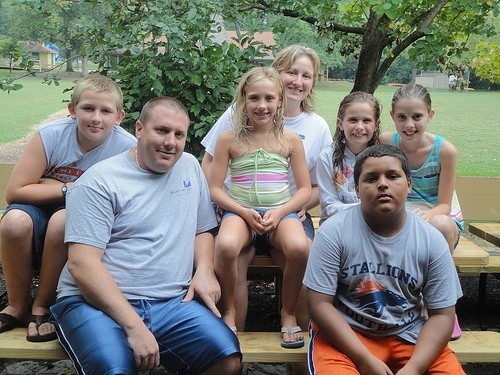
[61,182,68,199]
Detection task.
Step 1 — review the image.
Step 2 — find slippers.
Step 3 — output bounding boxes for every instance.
[230,326,237,337]
[25,314,57,342]
[0,313,26,333]
[281,326,304,348]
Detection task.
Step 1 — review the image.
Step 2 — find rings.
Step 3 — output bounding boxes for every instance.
[217,207,220,210]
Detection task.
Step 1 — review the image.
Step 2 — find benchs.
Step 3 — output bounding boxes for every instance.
[0,163,490,316]
[0,327,500,362]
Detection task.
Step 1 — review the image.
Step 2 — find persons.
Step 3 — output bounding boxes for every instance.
[48,97,243,375]
[0,75,138,342]
[315,91,382,227]
[378,83,464,341]
[200,45,334,375]
[208,66,312,348]
[302,143,467,375]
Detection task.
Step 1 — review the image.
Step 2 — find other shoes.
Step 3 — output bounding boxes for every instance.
[450,313,461,340]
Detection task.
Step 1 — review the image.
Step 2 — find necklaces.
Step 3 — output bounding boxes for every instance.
[135,147,140,166]
[346,145,368,156]
